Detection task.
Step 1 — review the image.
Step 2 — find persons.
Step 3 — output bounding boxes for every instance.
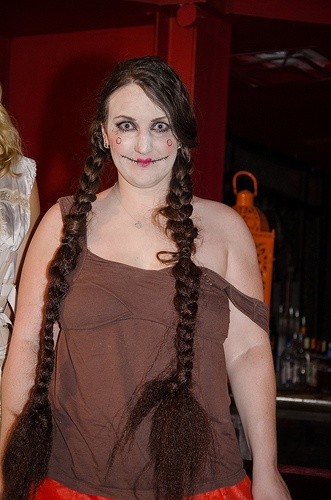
[0,55,291,500]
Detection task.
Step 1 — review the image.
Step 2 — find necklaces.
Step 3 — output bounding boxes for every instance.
[114,181,152,229]
